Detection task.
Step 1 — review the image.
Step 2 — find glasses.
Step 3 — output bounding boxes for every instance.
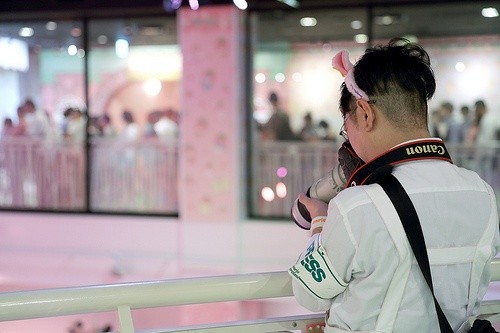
[338,99,376,140]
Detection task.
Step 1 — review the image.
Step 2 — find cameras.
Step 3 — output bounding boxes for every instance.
[291,140,367,230]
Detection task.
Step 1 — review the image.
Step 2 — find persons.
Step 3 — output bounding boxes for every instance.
[289,38,499,333]
[1,91,494,219]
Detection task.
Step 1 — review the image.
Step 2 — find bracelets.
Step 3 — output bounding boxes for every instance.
[309,215,327,234]
